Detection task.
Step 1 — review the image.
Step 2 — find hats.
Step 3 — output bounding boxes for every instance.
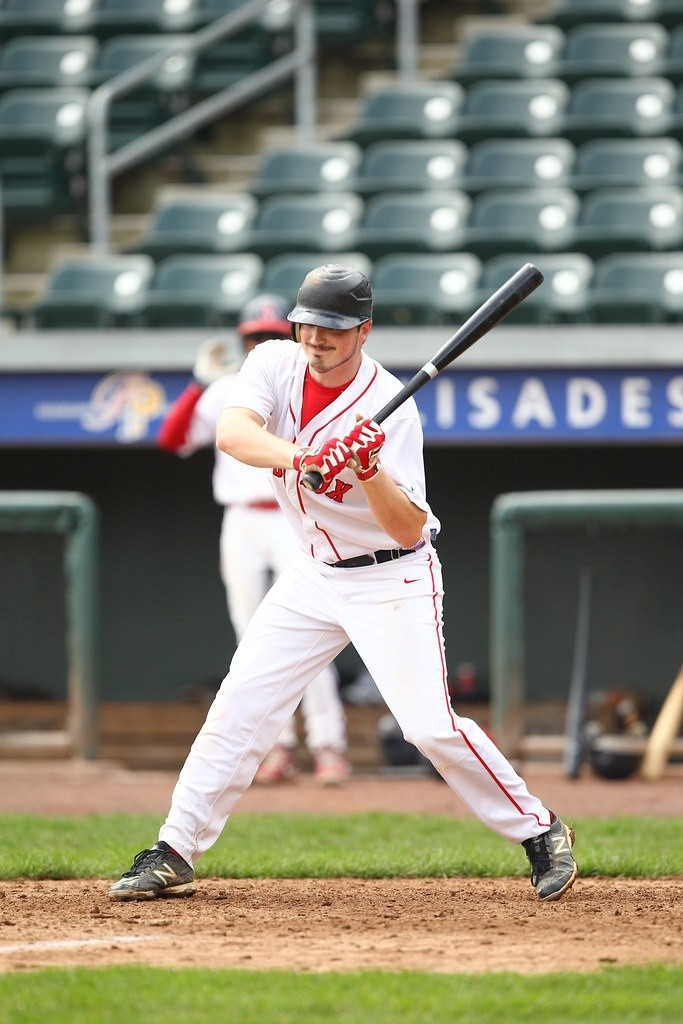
[234,292,291,335]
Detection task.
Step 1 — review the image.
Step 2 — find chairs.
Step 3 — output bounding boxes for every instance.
[0,0,683,325]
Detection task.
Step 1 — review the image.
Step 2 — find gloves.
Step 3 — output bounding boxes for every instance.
[345,412,386,482]
[292,438,352,483]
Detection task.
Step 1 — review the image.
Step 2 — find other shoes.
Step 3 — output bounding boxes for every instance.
[254,746,295,783]
[313,750,352,788]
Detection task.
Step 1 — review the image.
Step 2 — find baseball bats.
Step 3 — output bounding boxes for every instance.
[300,262,546,492]
[639,663,682,781]
[561,565,594,786]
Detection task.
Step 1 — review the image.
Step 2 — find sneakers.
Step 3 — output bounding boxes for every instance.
[520,810,577,900]
[108,842,197,899]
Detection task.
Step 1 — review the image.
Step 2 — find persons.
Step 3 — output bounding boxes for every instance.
[158,293,353,786]
[105,265,580,902]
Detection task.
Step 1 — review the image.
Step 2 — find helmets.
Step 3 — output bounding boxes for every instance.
[286,264,373,344]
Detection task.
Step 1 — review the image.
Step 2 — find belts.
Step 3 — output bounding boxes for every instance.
[324,548,416,568]
[244,498,278,510]
